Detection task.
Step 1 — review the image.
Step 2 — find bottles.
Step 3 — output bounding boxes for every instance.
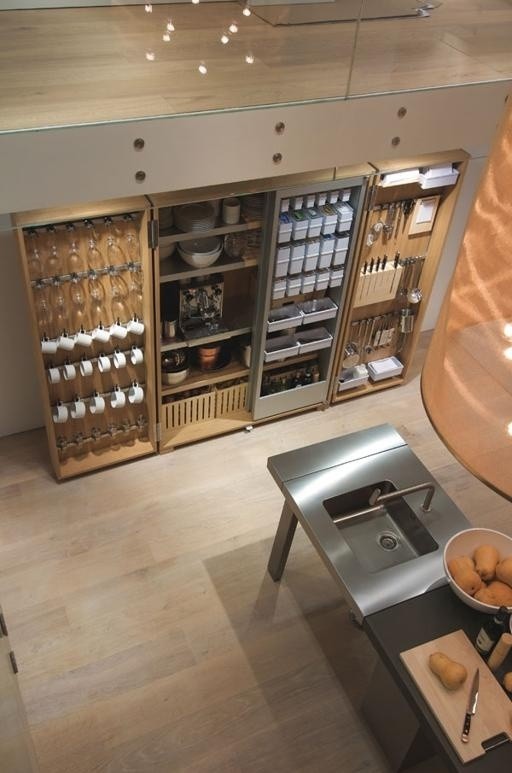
[475,604,510,659]
[262,365,321,397]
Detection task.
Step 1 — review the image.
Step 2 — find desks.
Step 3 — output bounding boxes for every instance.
[269,424,512,773]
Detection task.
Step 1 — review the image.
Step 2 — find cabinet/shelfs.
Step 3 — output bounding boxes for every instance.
[9,150,471,484]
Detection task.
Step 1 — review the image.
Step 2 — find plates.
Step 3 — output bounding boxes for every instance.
[173,201,217,234]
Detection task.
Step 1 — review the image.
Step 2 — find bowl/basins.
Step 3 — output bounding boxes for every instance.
[443,527,512,614]
[176,239,224,269]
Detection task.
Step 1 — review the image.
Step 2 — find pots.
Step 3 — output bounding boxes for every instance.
[160,341,224,386]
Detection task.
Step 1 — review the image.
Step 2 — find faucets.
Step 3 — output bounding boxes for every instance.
[370,482,436,512]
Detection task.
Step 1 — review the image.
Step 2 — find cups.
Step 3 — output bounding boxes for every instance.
[222,197,241,225]
[41,315,145,424]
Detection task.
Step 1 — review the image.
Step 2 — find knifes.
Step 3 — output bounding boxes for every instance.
[459,667,480,743]
[363,251,401,274]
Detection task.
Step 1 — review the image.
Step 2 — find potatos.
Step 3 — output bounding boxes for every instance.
[504,672,512,693]
[428,652,468,690]
[449,543,512,607]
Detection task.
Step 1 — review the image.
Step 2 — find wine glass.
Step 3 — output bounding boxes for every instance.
[26,213,145,339]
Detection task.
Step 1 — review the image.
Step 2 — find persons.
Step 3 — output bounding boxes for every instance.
[198,289,208,311]
[210,287,221,311]
[182,293,194,314]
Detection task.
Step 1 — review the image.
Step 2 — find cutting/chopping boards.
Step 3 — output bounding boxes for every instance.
[399,629,511,764]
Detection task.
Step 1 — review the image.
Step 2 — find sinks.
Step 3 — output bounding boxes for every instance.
[322,479,440,573]
